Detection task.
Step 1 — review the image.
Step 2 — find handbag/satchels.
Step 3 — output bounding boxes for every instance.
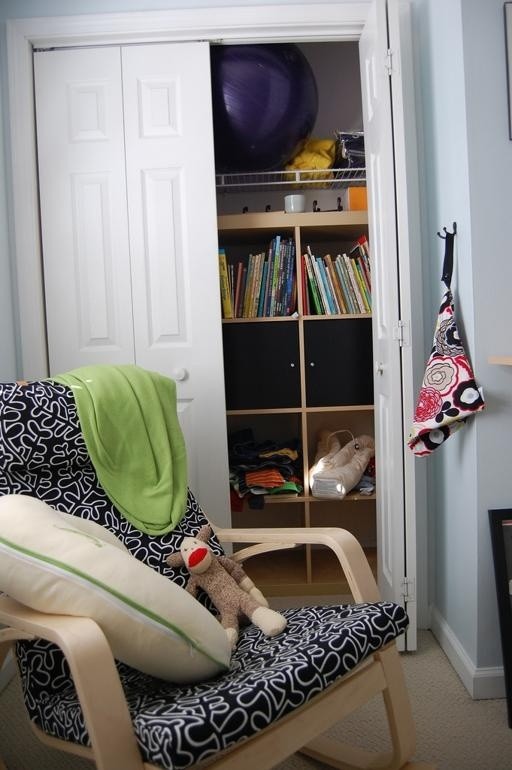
[408,231,486,458]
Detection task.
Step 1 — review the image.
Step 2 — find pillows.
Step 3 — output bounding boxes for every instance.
[0,490,230,686]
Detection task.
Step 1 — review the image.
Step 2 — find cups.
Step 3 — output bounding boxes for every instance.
[284,193,307,213]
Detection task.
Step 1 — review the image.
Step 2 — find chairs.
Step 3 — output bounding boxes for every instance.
[0,380,419,768]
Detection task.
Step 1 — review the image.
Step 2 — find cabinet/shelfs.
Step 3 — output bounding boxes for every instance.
[216,210,381,595]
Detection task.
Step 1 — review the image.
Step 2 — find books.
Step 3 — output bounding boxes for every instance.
[218,234,371,318]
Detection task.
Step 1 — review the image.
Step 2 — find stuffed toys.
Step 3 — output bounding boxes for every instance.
[166,524,287,656]
